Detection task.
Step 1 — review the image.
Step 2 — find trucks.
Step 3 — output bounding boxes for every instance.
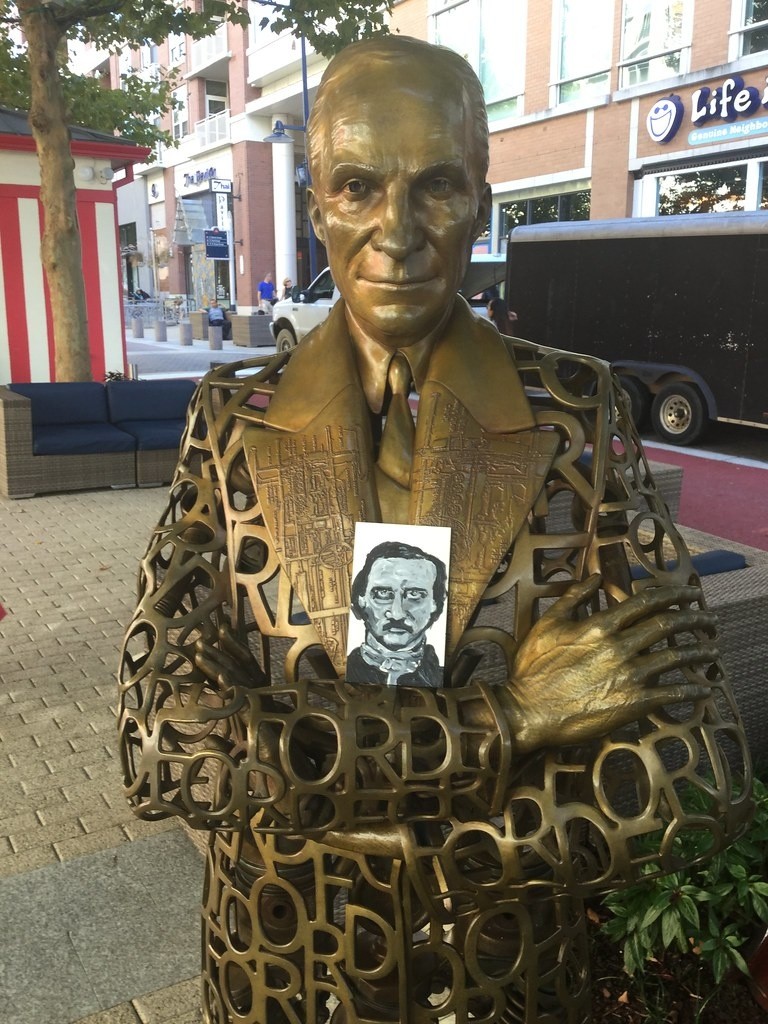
[504,211,768,444]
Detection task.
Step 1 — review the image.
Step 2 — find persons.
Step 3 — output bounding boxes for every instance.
[114,35,749,1012]
[257,273,279,312]
[281,278,295,299]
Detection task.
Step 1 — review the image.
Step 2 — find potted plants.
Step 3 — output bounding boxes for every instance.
[599,766,768,1011]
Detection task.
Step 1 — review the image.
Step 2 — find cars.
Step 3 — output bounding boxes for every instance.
[269,253,506,352]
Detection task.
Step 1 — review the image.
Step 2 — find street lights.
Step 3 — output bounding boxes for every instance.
[263,120,318,283]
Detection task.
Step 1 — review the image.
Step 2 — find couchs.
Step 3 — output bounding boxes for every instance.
[544,444,683,560]
[143,510,768,858]
[231,314,276,348]
[189,310,238,341]
[0,382,228,499]
